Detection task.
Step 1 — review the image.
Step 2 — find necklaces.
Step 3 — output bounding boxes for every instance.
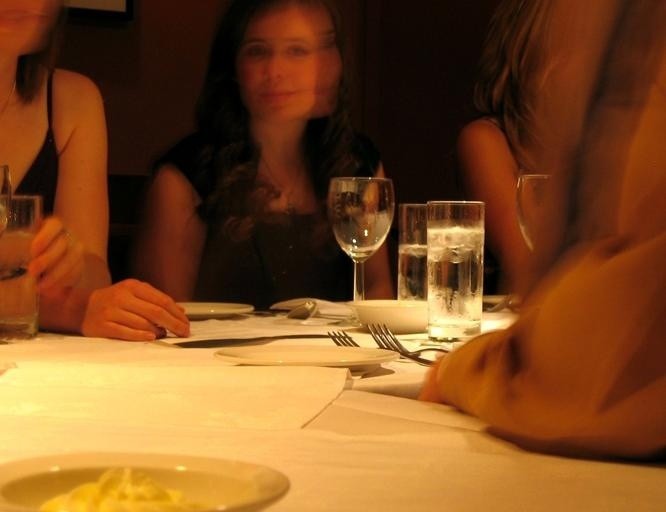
[256,151,302,216]
[0,81,16,117]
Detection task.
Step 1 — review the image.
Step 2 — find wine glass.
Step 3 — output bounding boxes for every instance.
[326,175,397,301]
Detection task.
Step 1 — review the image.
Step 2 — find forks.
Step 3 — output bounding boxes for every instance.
[323,322,452,368]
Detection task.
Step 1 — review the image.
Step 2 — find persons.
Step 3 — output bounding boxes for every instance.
[1,1,193,343]
[456,1,629,306]
[133,1,396,305]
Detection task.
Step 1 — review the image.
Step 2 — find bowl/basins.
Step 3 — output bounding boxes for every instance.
[344,293,430,333]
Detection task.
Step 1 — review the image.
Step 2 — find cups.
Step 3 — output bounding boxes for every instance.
[514,173,552,259]
[1,190,44,347]
[2,450,293,511]
[398,200,485,341]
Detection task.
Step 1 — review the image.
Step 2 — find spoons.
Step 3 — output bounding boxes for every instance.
[247,299,318,320]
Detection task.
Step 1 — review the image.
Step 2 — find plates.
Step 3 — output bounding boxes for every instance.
[211,342,402,376]
[171,301,257,321]
